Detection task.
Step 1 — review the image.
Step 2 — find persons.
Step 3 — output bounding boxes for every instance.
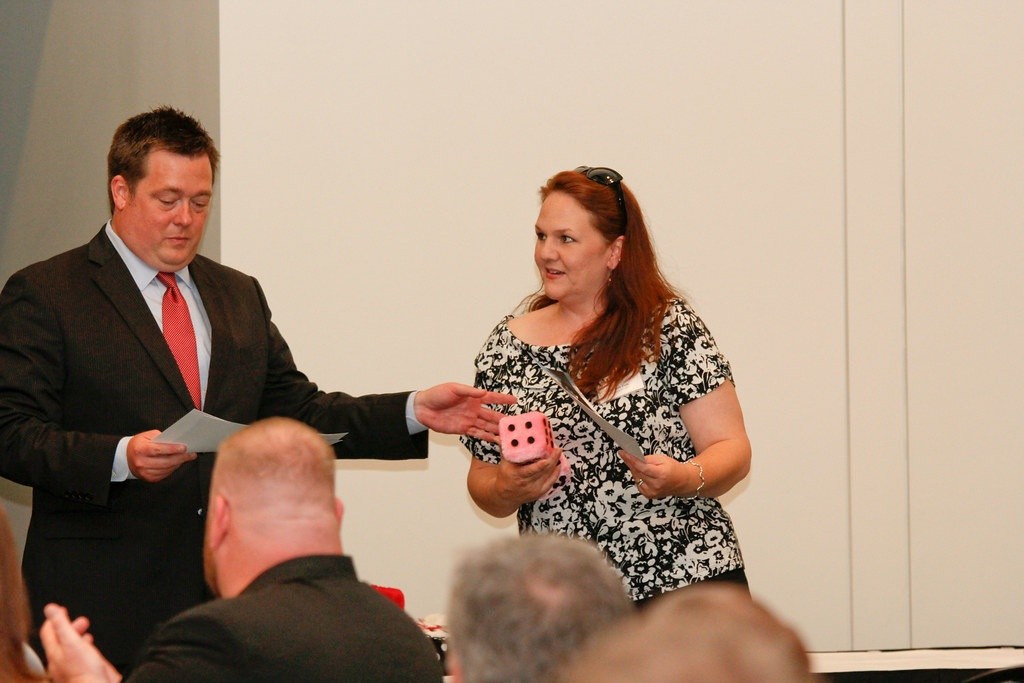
[458,163,752,609]
[37,416,443,683]
[0,104,518,680]
[566,583,811,683]
[444,536,634,683]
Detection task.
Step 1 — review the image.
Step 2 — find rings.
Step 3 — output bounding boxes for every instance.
[635,479,642,488]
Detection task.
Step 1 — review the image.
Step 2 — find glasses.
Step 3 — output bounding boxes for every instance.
[573,165,629,235]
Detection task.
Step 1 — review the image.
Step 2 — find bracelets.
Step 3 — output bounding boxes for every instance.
[676,458,706,504]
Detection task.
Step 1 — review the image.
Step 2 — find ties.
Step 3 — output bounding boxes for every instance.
[155,271,202,412]
